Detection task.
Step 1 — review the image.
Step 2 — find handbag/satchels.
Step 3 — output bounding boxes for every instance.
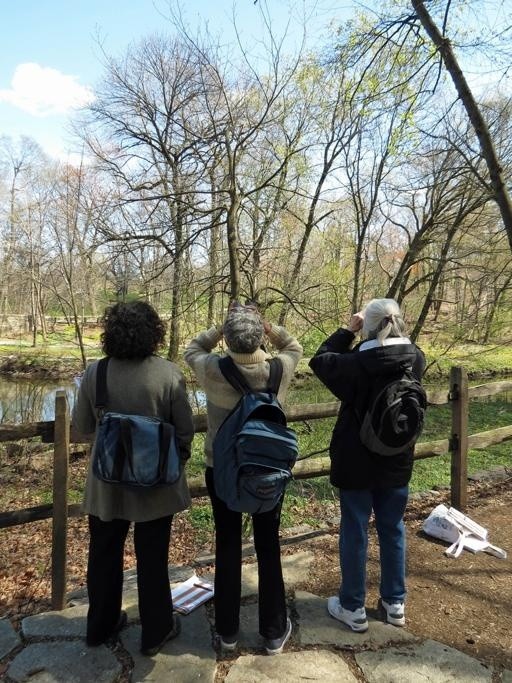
[422,503,508,562]
[88,410,187,491]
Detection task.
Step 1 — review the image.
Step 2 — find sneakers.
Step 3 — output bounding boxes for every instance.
[141,612,182,658]
[381,597,406,628]
[263,614,293,655]
[219,632,241,653]
[327,594,369,633]
[88,608,128,647]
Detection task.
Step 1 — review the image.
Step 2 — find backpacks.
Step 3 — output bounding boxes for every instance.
[209,353,301,517]
[350,345,430,464]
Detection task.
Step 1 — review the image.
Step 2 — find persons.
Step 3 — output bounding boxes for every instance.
[311,298,426,634]
[70,299,194,654]
[186,303,292,657]
